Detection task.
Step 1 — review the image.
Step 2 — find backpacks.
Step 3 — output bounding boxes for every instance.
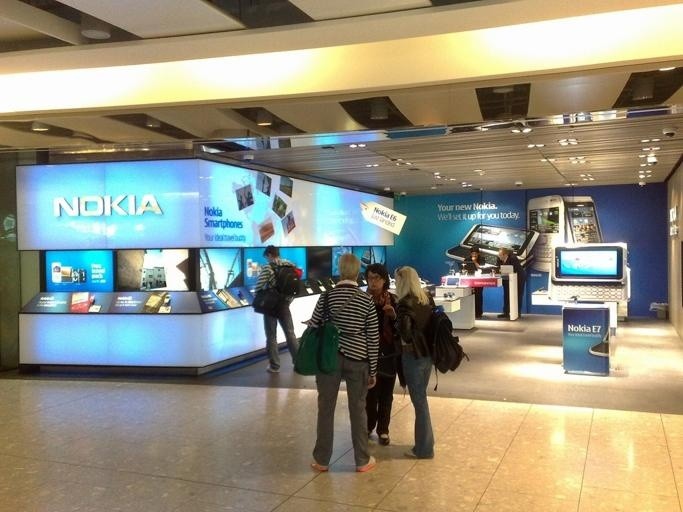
[425,291,465,374]
[269,261,300,297]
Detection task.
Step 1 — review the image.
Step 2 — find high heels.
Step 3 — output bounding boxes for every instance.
[368,430,390,446]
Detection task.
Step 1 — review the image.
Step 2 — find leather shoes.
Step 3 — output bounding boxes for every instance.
[497,313,521,320]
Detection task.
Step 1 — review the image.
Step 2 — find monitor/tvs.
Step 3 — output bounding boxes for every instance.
[446,276,460,287]
[501,265,513,274]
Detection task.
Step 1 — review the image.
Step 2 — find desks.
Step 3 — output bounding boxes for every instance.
[388,266,631,378]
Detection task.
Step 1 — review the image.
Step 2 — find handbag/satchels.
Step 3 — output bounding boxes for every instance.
[252,288,281,316]
[293,321,338,376]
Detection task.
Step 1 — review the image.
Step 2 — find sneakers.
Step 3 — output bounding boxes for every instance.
[310,463,328,471]
[356,456,376,472]
[267,364,279,373]
[404,446,434,459]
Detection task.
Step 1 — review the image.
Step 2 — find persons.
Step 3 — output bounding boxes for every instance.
[492,247,526,319]
[254,245,300,373]
[460,244,485,319]
[364,264,397,445]
[390,265,436,459]
[306,253,377,471]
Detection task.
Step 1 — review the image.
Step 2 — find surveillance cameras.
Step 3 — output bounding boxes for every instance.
[515,183,522,187]
[244,155,254,163]
[664,128,677,136]
[648,157,658,166]
[639,181,647,188]
[400,192,406,197]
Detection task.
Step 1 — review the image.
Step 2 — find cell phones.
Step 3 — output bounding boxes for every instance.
[563,196,602,243]
[527,195,565,273]
[446,245,534,272]
[549,246,629,302]
[460,224,539,260]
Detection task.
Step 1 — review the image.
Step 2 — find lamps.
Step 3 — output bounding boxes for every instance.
[79,14,112,40]
[146,117,160,127]
[31,122,49,131]
[256,110,272,126]
[370,100,389,120]
